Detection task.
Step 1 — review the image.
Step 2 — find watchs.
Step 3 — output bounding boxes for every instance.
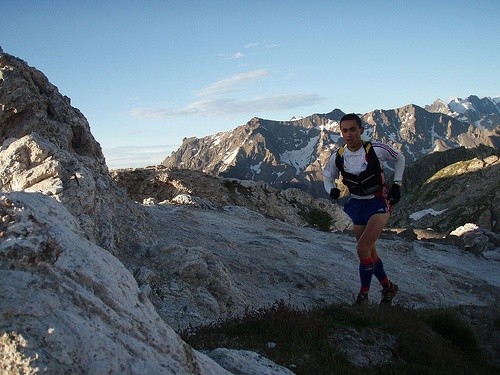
[394,181,402,186]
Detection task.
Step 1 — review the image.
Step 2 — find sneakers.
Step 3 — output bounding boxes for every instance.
[352,291,368,307]
[380,280,399,306]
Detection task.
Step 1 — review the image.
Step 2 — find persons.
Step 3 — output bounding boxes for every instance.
[322,113,407,306]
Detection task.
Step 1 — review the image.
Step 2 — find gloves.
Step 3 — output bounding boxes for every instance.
[330,188,341,200]
[387,183,401,206]
